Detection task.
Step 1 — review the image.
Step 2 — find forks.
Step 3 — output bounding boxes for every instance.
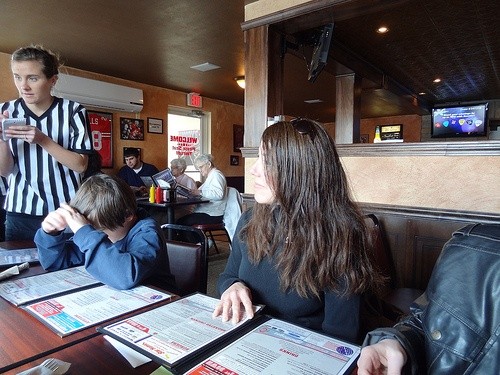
[25,358,60,375]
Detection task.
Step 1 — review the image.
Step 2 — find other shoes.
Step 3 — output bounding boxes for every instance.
[196,239,212,250]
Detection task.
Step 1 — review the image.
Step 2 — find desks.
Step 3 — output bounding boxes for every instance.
[137,196,210,240]
[0,239,358,375]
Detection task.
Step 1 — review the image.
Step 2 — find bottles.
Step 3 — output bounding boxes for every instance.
[149,184,170,204]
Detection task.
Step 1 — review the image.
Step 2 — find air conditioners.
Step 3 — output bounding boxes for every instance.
[51,73,143,112]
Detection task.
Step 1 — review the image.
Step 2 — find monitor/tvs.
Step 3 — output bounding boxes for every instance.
[307,22,336,83]
[380,124,403,141]
[431,101,489,138]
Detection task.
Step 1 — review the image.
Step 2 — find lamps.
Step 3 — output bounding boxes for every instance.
[233,75,245,89]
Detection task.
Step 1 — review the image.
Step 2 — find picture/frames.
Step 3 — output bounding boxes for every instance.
[123,147,141,164]
[119,117,144,141]
[86,110,114,169]
[233,124,244,152]
[147,117,163,135]
[229,155,240,166]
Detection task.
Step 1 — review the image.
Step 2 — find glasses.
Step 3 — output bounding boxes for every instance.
[291,116,315,143]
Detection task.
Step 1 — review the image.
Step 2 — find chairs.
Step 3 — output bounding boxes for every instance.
[189,188,239,255]
[160,223,210,297]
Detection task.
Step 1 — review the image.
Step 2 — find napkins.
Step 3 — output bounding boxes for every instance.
[17,358,71,375]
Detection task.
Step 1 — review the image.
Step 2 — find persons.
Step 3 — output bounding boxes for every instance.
[170,158,197,223]
[117,147,159,195]
[0,43,94,241]
[356,223,500,375]
[33,173,178,291]
[213,118,391,348]
[175,153,228,248]
[84,150,103,178]
